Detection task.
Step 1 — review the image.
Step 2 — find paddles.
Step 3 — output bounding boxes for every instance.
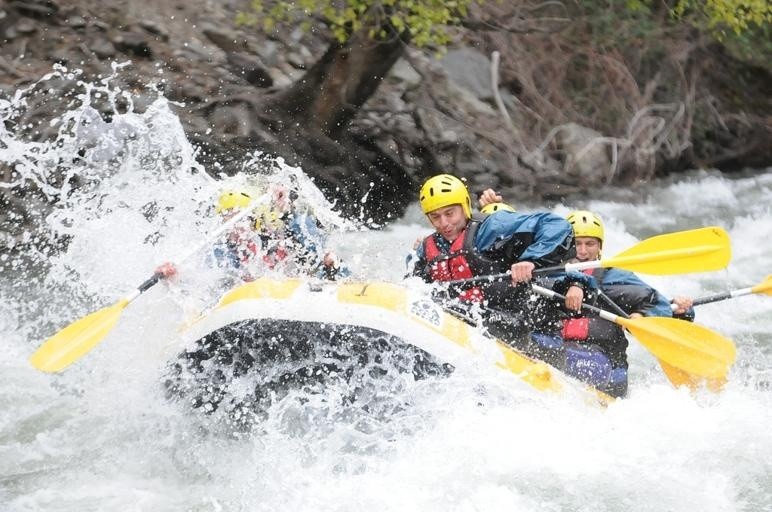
[434,227,731,286]
[30,194,274,373]
[669,275,770,308]
[524,282,738,392]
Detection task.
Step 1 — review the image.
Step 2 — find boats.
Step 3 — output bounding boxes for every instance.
[162,276,619,458]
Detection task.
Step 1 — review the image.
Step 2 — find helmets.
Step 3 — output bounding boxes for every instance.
[480,202,515,215]
[214,192,280,238]
[564,209,605,260]
[419,173,472,219]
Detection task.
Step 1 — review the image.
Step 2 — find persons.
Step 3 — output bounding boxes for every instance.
[153,186,352,306]
